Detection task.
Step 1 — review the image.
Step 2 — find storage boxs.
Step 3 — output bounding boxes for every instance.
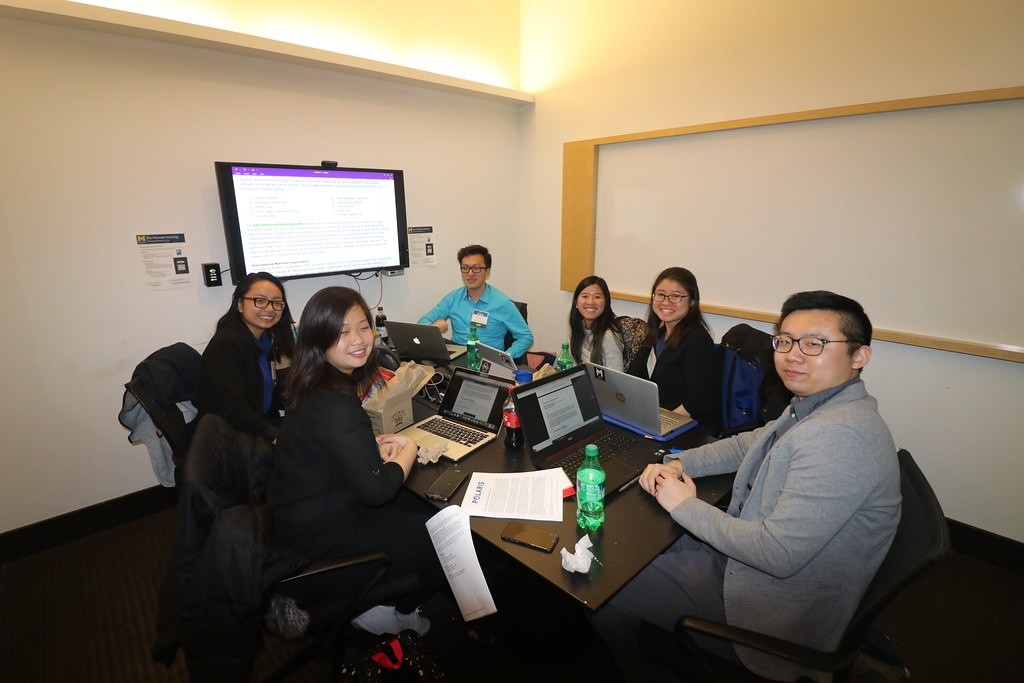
[363,361,435,434]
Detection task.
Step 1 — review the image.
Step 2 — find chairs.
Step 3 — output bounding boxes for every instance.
[125,302,950,683]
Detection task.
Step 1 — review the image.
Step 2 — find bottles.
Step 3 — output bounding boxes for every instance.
[576,444,605,532]
[502,385,524,449]
[556,343,573,372]
[375,307,389,345]
[467,327,482,371]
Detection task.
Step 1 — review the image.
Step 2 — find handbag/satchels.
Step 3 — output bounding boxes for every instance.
[715,342,765,431]
[339,629,447,683]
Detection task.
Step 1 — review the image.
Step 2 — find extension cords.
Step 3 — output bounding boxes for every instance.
[414,392,441,411]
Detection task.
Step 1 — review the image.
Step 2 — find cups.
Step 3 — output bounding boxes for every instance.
[513,369,533,387]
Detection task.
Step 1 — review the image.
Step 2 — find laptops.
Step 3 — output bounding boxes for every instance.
[381,321,693,504]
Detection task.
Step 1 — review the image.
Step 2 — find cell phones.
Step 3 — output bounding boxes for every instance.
[427,467,469,501]
[502,521,559,553]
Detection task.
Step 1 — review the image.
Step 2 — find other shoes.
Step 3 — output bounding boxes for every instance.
[264,590,309,640]
[352,605,431,638]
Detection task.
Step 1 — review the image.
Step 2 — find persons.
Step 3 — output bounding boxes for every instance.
[189,272,295,446]
[627,266,716,418]
[266,286,441,637]
[567,276,625,372]
[585,291,902,683]
[418,245,535,358]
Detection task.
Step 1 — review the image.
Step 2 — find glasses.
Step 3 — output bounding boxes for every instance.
[460,266,487,273]
[242,296,286,311]
[771,335,864,357]
[651,292,692,303]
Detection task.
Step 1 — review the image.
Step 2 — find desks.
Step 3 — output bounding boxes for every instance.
[348,339,732,616]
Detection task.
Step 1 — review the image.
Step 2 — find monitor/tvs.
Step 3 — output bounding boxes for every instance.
[214,161,410,286]
